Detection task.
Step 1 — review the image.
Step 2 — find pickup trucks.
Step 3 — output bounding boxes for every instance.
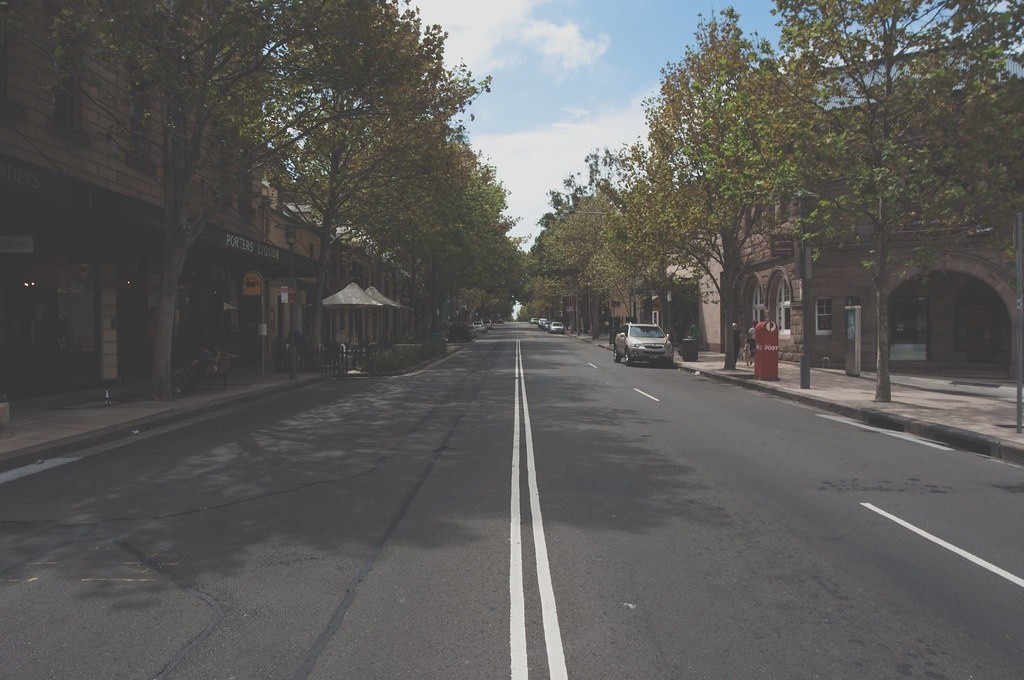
[549,321,565,334]
[530,317,549,330]
[472,321,487,333]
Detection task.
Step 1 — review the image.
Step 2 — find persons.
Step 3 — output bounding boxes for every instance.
[675,320,699,356]
[190,343,232,377]
[732,320,758,367]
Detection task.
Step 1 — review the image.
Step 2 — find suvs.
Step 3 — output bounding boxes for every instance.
[613,322,674,367]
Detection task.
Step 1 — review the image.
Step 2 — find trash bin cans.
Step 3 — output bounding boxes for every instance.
[607,330,618,344]
[681,338,699,362]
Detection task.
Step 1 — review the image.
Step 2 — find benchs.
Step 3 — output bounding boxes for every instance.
[183,353,239,396]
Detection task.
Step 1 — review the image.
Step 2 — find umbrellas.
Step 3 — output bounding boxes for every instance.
[304,283,399,346]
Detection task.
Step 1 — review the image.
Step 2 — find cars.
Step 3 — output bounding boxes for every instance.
[497,318,503,324]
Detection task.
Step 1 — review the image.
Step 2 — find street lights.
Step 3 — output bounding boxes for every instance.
[274,222,306,378]
[795,192,822,388]
